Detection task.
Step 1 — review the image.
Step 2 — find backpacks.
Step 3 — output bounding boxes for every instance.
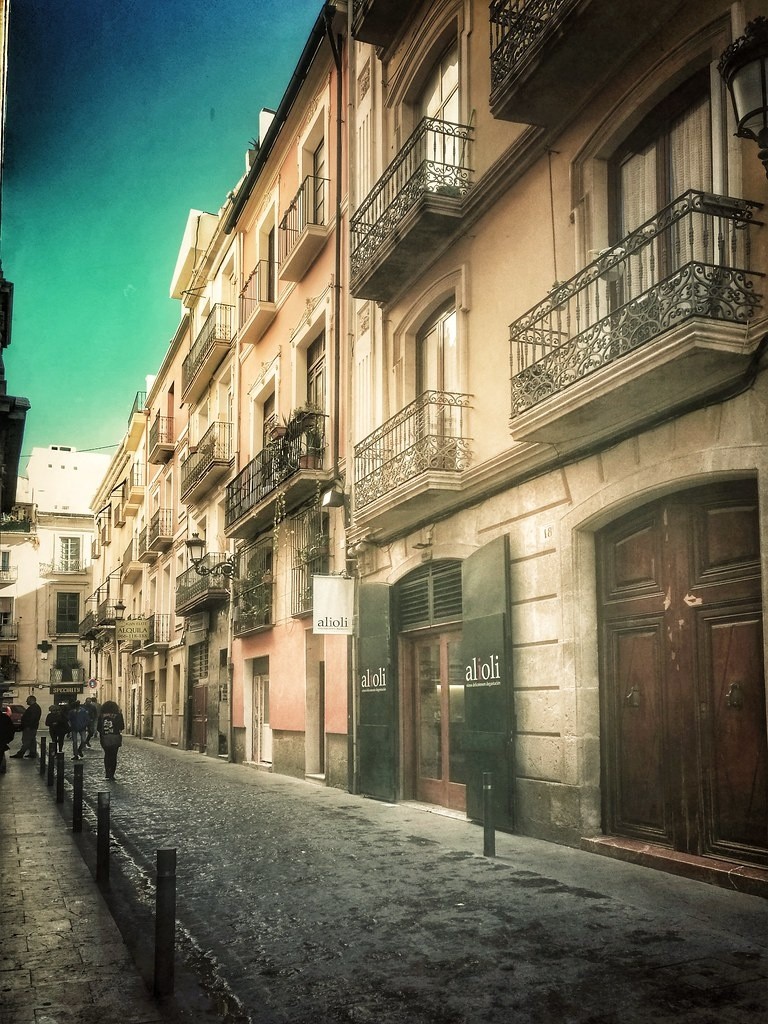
[103,716,118,734]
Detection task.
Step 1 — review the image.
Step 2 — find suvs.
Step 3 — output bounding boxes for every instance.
[1,703,27,732]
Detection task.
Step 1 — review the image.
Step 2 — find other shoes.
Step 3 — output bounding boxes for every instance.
[10,754,23,758]
[72,756,78,760]
[78,750,83,757]
[25,754,36,758]
[85,740,91,747]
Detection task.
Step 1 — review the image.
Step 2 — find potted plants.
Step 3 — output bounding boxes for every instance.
[264,401,325,483]
[235,572,274,628]
[50,659,66,683]
[68,660,83,682]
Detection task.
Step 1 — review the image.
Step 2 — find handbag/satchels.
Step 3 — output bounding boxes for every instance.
[101,734,122,751]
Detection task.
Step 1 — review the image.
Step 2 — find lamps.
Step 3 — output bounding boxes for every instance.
[716,16,768,181]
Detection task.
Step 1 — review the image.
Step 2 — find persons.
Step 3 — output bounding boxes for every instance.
[9,695,41,758]
[96,701,125,781]
[44,697,101,760]
[0,711,14,766]
